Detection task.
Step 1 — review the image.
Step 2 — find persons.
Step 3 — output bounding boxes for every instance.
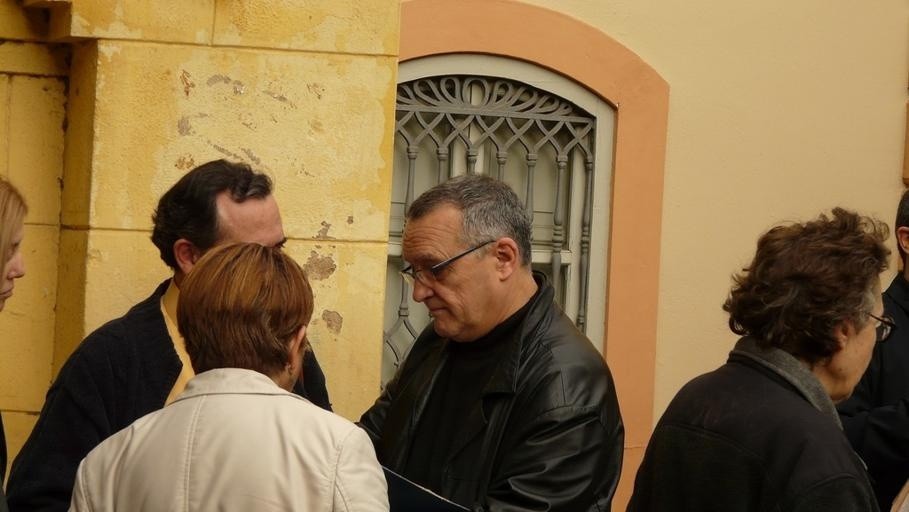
[829,190,909,512]
[624,207,894,512]
[66,242,390,512]
[354,172,624,512]
[4,160,333,511]
[0,175,29,512]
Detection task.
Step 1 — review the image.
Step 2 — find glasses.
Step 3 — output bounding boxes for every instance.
[399,240,495,289]
[847,306,895,344]
